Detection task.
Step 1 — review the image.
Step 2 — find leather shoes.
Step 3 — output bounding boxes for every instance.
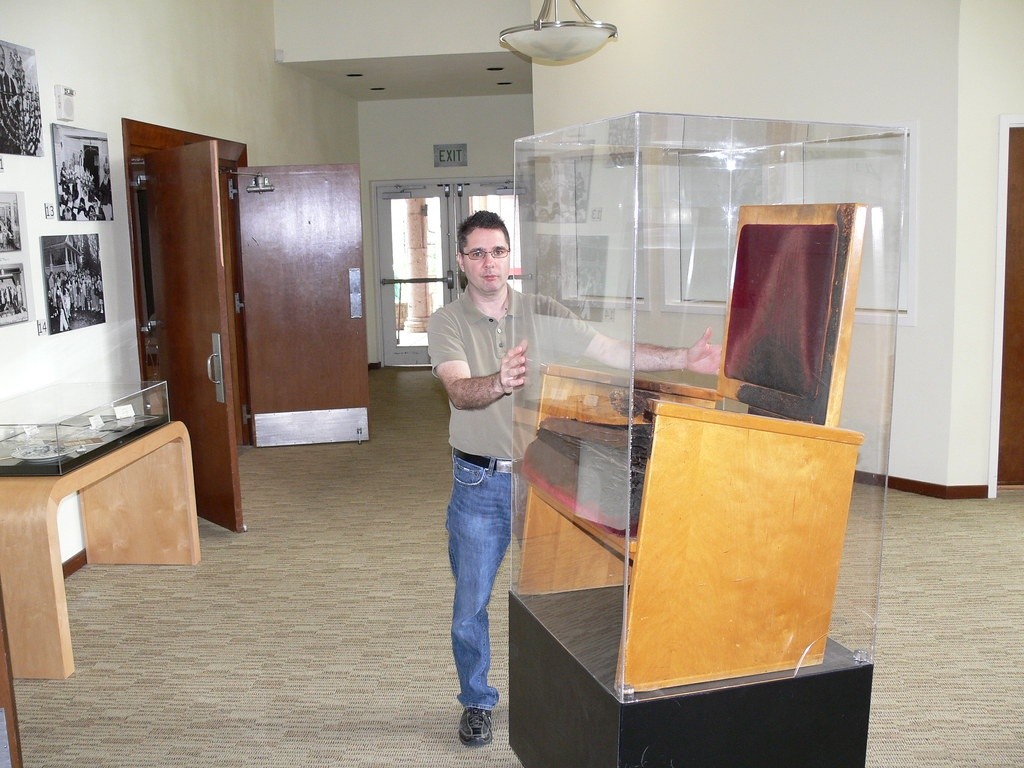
[458,706,494,746]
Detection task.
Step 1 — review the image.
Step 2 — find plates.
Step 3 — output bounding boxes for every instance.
[10,444,78,464]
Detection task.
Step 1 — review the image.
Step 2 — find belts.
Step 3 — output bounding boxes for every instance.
[453,448,523,475]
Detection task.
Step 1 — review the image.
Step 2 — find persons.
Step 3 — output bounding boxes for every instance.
[0,147,111,330]
[423,211,721,744]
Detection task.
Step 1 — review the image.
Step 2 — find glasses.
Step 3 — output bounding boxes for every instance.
[459,247,511,260]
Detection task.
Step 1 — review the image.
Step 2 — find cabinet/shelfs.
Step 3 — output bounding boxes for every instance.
[504,112,914,766]
[1,376,201,680]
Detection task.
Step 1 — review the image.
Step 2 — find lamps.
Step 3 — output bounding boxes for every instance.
[497,1,616,67]
